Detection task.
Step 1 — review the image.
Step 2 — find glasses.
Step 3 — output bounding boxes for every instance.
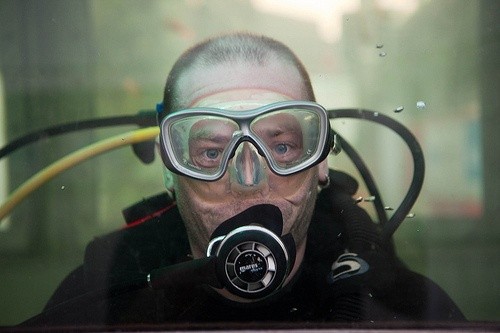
[159,100,331,180]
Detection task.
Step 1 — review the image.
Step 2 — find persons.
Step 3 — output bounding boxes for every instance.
[5,32,468,329]
